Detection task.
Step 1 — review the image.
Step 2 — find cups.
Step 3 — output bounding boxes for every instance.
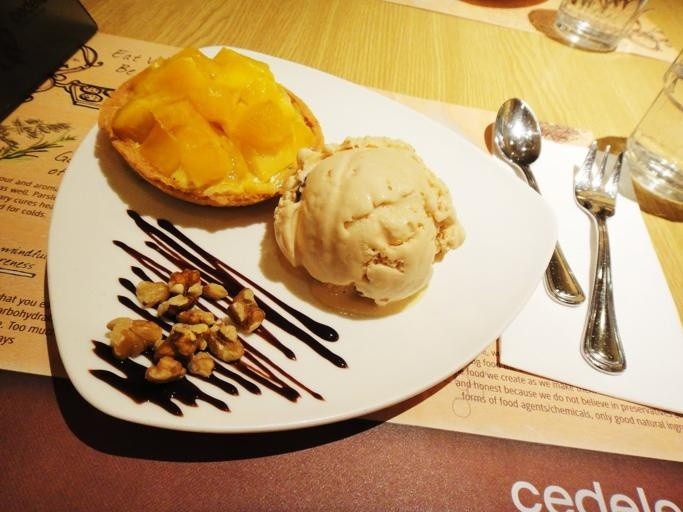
[551,1,645,50]
[629,39,682,203]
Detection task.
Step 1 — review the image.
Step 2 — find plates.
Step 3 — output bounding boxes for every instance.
[43,44,566,435]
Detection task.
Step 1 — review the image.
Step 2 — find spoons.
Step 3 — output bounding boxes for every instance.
[494,98,587,302]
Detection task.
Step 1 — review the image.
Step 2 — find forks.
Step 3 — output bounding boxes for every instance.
[577,141,624,372]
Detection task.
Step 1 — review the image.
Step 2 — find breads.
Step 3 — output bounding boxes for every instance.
[100,47,324,210]
